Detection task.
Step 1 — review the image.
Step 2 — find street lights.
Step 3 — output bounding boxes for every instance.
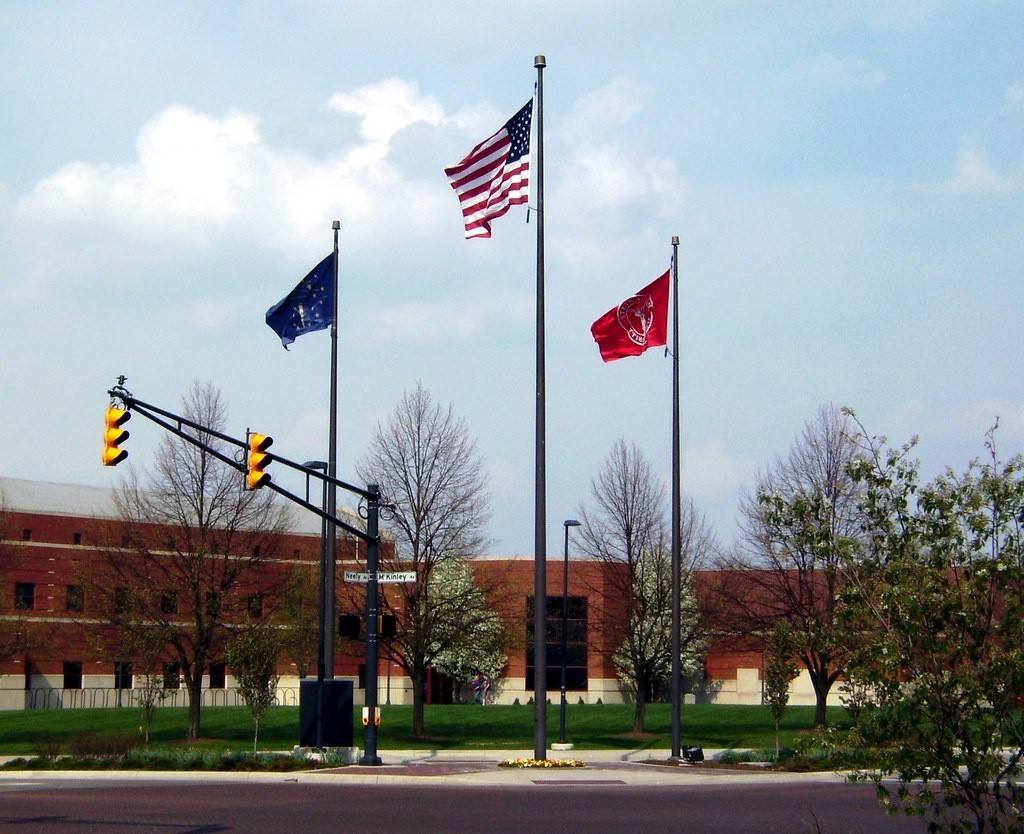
[302,460,327,679]
[550,520,581,753]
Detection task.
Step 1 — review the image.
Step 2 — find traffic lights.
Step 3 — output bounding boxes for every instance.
[247,434,274,490]
[101,407,131,468]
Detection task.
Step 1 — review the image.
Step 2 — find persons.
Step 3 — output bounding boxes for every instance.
[472,674,493,705]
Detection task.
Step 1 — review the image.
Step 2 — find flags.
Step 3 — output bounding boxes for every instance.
[444,97,533,240]
[590,269,672,362]
[265,251,336,351]
